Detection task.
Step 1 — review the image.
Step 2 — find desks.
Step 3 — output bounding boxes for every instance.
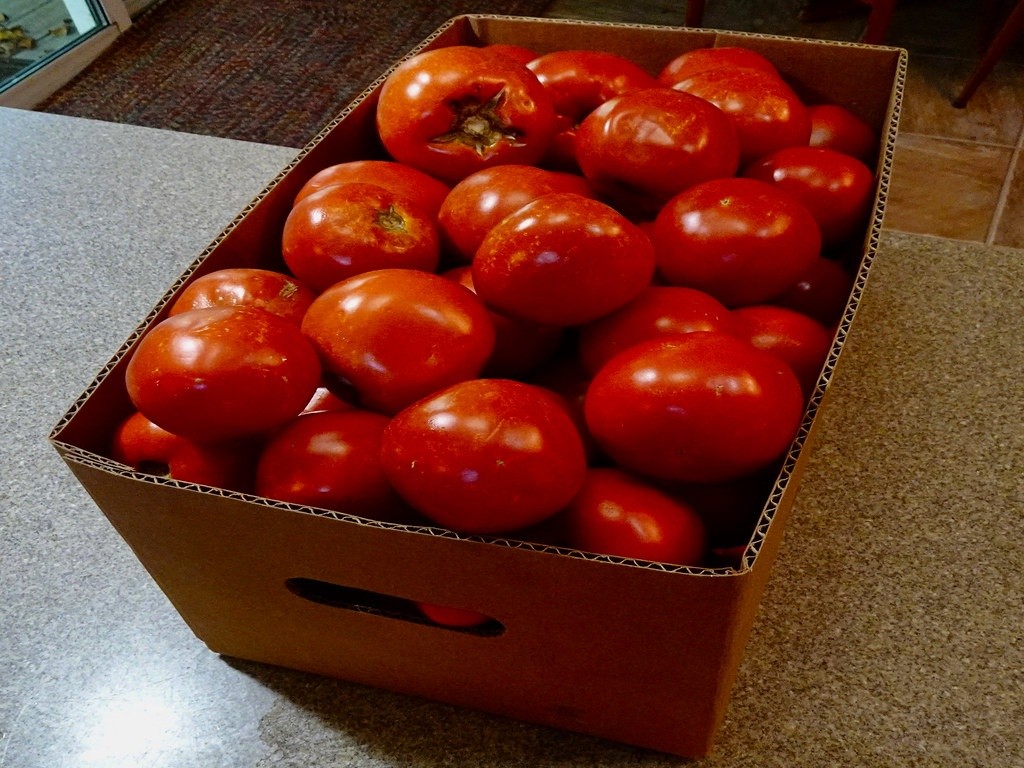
[0,105,1024,766]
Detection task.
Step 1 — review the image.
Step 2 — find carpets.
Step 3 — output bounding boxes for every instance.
[32,0,549,147]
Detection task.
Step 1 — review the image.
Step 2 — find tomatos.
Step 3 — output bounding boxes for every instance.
[112,43,877,631]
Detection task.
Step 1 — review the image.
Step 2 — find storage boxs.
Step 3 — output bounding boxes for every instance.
[49,13,911,760]
